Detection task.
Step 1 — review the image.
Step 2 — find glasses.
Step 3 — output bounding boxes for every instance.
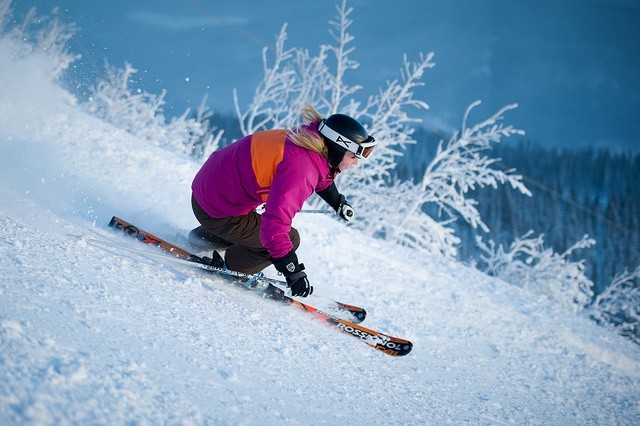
[318,121,376,160]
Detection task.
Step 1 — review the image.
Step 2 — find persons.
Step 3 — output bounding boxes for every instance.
[191,111,369,297]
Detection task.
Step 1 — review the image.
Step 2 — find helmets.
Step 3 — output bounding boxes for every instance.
[317,114,368,168]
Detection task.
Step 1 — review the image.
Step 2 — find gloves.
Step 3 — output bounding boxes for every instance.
[315,181,354,221]
[270,248,309,297]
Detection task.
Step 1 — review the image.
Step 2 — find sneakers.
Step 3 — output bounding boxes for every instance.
[224,264,270,291]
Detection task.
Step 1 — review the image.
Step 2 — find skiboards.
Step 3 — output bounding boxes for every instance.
[108,216,413,357]
